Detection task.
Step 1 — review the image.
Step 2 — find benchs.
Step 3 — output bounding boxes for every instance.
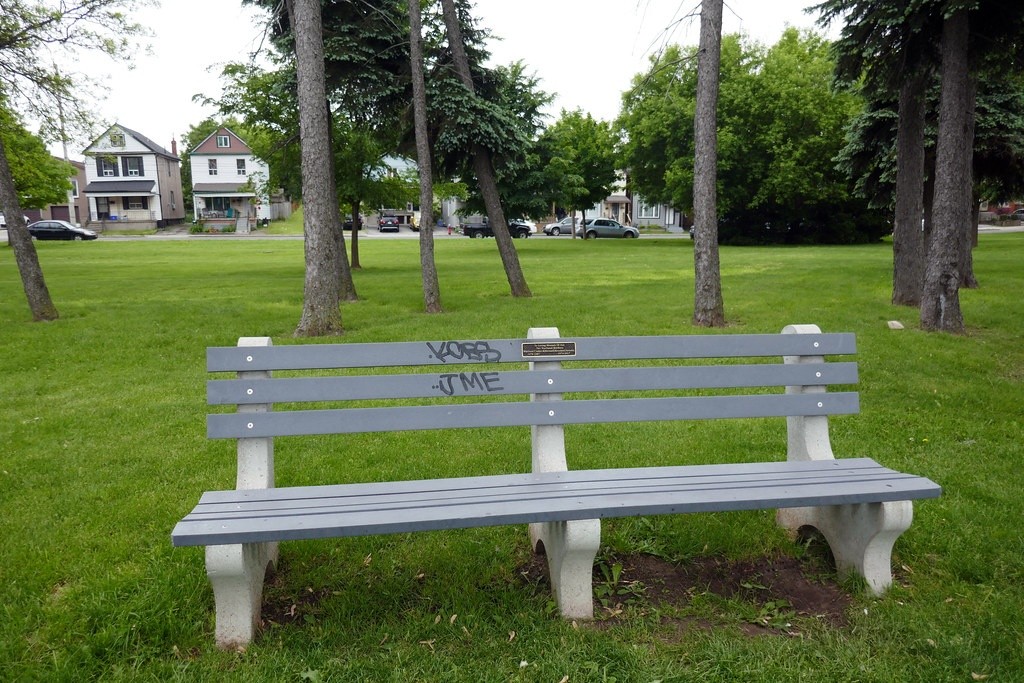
[171,322,942,650]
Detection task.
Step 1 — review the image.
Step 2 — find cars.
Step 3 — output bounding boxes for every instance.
[344,214,363,231]
[27,220,98,241]
[510,218,537,235]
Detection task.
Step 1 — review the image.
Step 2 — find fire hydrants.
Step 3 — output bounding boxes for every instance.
[448,225,451,235]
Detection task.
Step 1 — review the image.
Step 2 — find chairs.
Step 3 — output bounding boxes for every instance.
[201,206,234,218]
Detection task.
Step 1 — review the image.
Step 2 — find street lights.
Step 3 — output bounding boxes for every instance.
[35,40,76,227]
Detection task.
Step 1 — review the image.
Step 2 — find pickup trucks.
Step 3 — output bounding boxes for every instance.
[458,217,532,239]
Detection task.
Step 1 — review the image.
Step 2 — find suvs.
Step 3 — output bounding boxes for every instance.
[378,216,399,232]
[409,213,421,230]
[576,218,640,239]
[543,218,579,236]
[0,211,32,227]
